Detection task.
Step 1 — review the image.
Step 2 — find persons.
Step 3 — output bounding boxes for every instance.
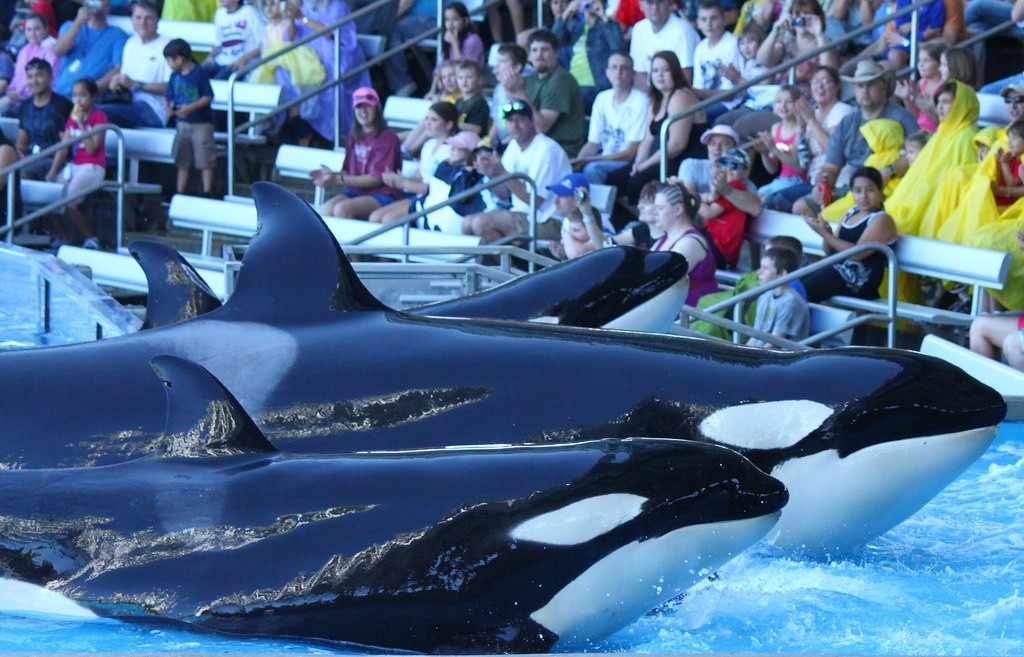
[0,0,1024,370]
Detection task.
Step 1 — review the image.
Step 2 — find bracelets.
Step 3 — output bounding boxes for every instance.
[339,174,346,184]
[136,82,145,92]
[635,163,641,173]
[903,39,910,50]
[583,216,595,223]
[300,17,308,25]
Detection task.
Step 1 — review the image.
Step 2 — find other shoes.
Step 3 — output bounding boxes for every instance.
[160,197,172,206]
[84,236,98,250]
[50,238,70,247]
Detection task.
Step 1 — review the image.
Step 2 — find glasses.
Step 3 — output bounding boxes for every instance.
[811,76,836,86]
[717,158,743,171]
[502,101,528,114]
[1005,95,1024,103]
[355,93,379,102]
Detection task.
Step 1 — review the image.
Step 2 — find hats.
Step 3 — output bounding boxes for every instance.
[472,137,503,155]
[443,130,480,152]
[1000,81,1024,98]
[700,124,739,147]
[714,148,749,171]
[840,60,896,99]
[546,173,590,198]
[352,88,382,109]
[500,98,534,119]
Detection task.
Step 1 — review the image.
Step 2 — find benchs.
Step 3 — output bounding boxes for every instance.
[1,18,1024,394]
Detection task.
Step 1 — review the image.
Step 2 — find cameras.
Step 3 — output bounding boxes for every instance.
[575,188,587,201]
[579,0,594,12]
[790,16,805,27]
